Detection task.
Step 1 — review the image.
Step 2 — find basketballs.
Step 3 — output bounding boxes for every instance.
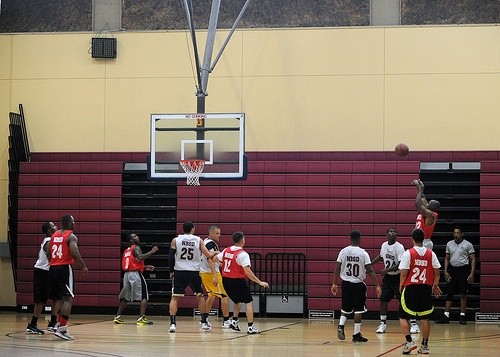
[394,143,409,156]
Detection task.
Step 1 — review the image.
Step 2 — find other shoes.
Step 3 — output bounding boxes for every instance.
[402,341,417,354]
[247,325,261,334]
[409,318,420,334]
[199,318,212,327]
[136,314,153,326]
[168,324,176,332]
[229,319,242,332]
[336,325,345,340]
[201,322,212,332]
[417,344,430,354]
[351,332,368,342]
[376,324,387,334]
[435,314,449,324]
[113,314,126,324]
[222,318,232,328]
[459,315,467,325]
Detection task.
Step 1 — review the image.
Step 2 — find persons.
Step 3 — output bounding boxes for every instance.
[435,227,476,324]
[168,220,222,332]
[330,230,382,342]
[113,233,159,324]
[212,232,269,334]
[370,227,421,334]
[46,214,89,340]
[398,228,442,353]
[26,222,58,335]
[200,224,233,328]
[410,178,440,251]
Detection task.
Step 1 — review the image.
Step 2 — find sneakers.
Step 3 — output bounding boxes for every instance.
[26,322,44,335]
[47,319,55,331]
[54,326,74,341]
[53,322,60,332]
